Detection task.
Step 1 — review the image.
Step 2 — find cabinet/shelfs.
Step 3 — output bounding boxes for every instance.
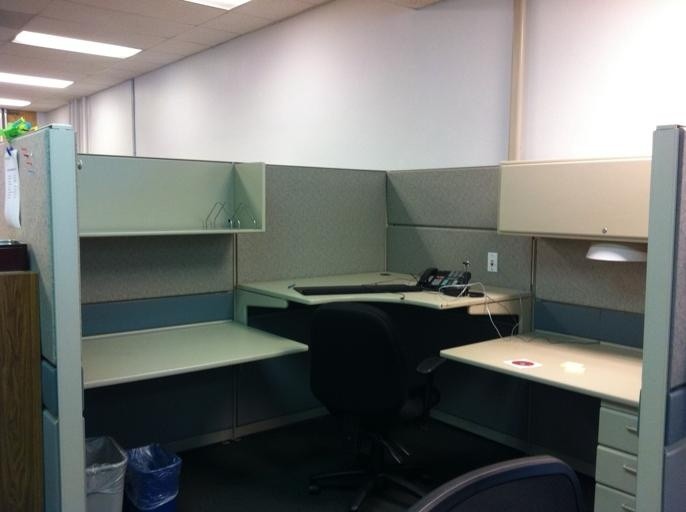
[497,162,651,240]
[594,403,642,512]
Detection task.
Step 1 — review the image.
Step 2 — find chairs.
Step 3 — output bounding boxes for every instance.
[311,302,448,512]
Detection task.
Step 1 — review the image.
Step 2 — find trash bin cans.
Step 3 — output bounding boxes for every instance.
[85,436,128,512]
[124,443,182,512]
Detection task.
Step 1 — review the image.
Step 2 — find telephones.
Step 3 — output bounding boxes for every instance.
[416,267,471,297]
